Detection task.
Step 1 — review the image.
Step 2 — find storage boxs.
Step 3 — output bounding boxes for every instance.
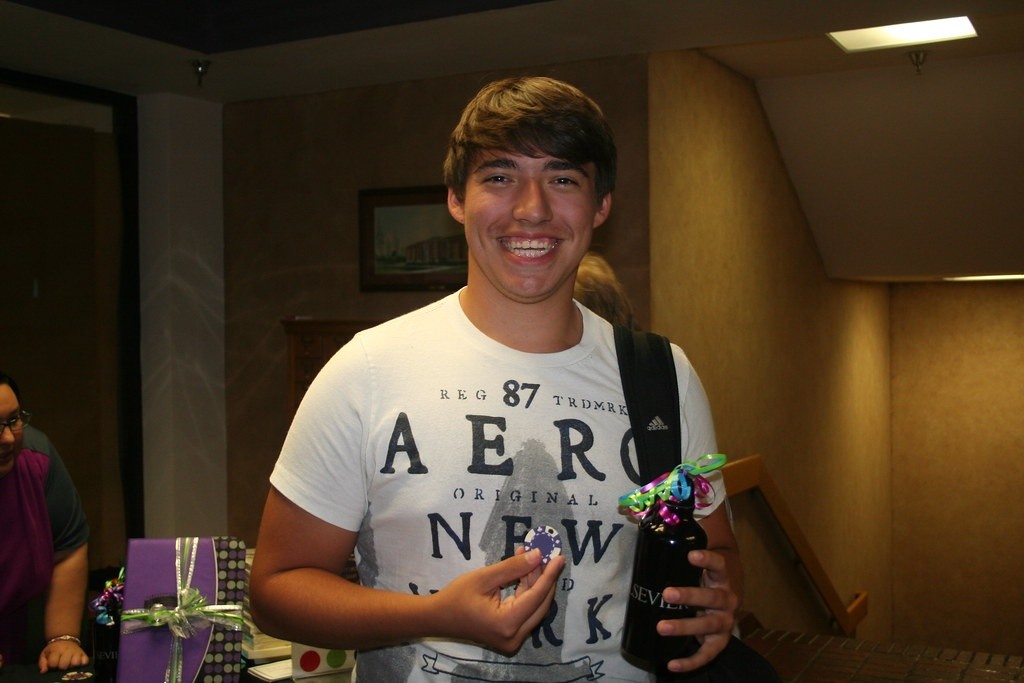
[116,537,248,683]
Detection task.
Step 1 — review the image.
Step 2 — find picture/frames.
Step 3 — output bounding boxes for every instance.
[354,186,473,292]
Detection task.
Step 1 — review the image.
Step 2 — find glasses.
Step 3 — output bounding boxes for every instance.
[0,407,31,435]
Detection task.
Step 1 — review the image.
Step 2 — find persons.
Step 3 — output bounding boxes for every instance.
[573,249,622,324]
[247,69,741,682]
[0,378,92,673]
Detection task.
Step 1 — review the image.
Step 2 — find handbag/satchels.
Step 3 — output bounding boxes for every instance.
[617,326,780,682]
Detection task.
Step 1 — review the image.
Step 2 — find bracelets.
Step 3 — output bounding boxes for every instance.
[48,634,81,645]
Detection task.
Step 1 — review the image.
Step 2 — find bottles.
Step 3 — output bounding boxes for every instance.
[621,478,708,676]
[92,600,120,683]
